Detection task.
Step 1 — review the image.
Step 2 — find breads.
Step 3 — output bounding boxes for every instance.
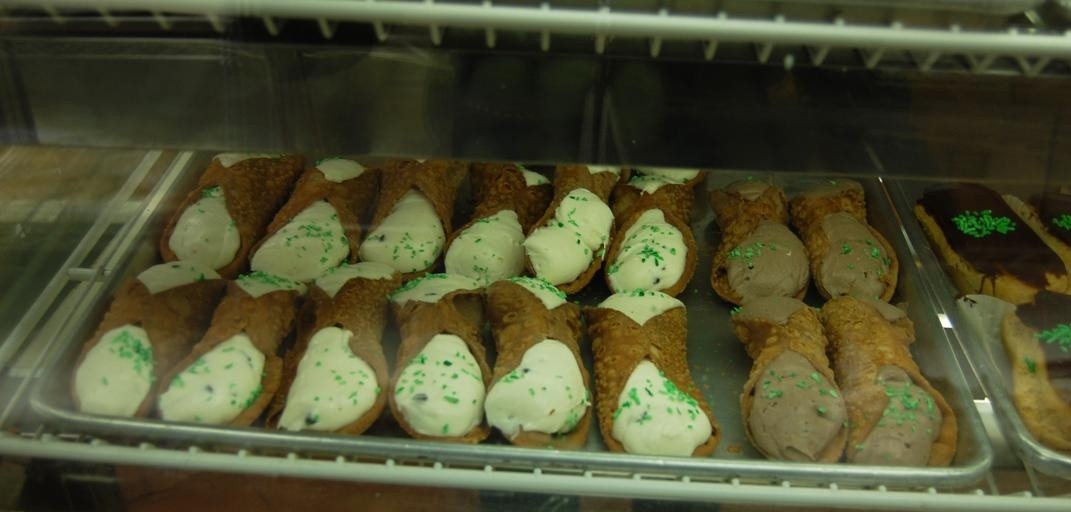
[906,189,1064,298]
[1006,187,1070,299]
[1007,299,1064,456]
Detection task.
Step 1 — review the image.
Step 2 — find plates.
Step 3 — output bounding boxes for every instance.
[21,148,998,494]
[890,134,1069,483]
